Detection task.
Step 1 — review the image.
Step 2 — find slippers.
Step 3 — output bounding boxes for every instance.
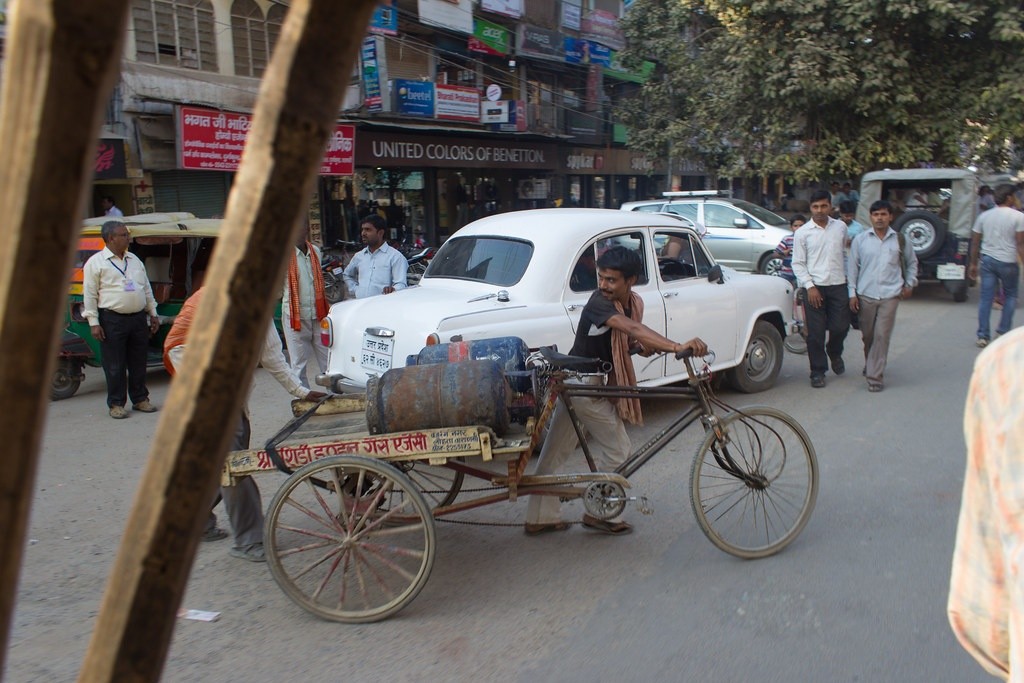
[201,528,226,542]
[229,543,281,562]
[581,515,633,535]
[525,520,571,536]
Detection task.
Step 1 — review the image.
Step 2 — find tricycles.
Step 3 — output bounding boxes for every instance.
[218,340,820,625]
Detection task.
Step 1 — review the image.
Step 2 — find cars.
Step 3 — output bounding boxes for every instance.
[323,203,795,403]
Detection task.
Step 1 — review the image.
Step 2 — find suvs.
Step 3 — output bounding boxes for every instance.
[620,188,797,277]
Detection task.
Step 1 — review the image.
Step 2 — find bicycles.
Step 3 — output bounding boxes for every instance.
[783,250,808,355]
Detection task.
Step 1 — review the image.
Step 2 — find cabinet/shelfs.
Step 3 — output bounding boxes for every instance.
[411,189,425,246]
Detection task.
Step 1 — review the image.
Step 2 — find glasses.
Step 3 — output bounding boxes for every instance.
[115,232,129,238]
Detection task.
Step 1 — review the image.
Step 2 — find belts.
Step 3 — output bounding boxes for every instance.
[102,309,144,316]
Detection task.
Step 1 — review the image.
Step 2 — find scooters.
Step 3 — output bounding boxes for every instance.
[45,212,287,401]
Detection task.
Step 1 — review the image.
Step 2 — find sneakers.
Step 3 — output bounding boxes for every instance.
[976,338,988,347]
[826,346,844,373]
[109,404,129,419]
[132,398,156,412]
[811,374,825,387]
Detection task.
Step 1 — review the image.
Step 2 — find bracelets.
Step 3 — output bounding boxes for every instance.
[673,343,681,352]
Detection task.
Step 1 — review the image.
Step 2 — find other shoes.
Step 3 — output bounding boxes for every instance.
[862,365,883,391]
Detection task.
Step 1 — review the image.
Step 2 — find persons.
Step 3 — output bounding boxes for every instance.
[791,190,850,387]
[660,211,703,265]
[773,215,806,326]
[473,178,484,217]
[340,176,361,243]
[455,175,474,227]
[164,287,329,562]
[277,220,335,399]
[947,325,1024,683]
[356,173,373,226]
[524,246,710,536]
[759,192,795,211]
[81,221,159,417]
[847,200,919,392]
[484,176,499,215]
[344,215,409,299]
[967,181,1024,347]
[829,182,849,218]
[842,182,860,202]
[892,189,946,209]
[102,196,122,217]
[837,200,864,248]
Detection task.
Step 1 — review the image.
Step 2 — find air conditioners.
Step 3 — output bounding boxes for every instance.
[518,179,549,199]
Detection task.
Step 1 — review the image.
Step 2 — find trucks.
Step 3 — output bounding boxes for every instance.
[856,166,1018,304]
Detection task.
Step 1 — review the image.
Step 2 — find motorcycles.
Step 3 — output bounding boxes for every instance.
[321,247,345,303]
[393,238,439,286]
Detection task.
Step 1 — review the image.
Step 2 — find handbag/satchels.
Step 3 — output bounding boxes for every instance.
[895,230,908,281]
